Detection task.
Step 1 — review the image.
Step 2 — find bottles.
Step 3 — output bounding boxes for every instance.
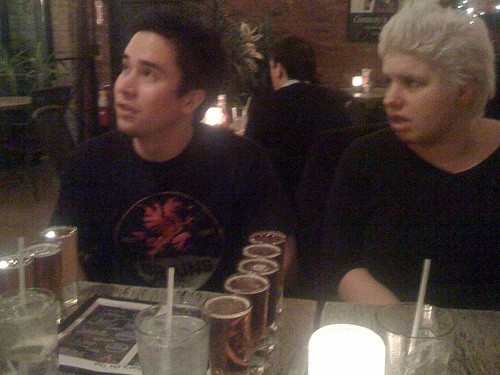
[216,94,231,129]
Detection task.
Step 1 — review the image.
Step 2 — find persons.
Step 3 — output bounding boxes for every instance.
[245,35,353,188]
[48,5,300,294]
[328,0,500,311]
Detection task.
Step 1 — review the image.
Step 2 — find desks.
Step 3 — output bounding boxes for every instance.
[77,280,318,375]
[319,299,500,375]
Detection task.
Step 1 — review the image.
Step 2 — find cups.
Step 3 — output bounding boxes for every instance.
[40,226,81,308]
[0,253,34,296]
[248,232,286,314]
[243,244,283,333]
[17,243,65,324]
[232,106,249,136]
[134,303,212,375]
[224,272,270,375]
[238,258,279,352]
[0,287,61,375]
[362,68,371,92]
[201,295,254,375]
[374,301,457,375]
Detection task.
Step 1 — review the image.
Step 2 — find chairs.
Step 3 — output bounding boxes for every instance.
[294,120,391,297]
[0,84,75,201]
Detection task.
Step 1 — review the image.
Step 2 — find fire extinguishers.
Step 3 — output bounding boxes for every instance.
[95,84,112,128]
